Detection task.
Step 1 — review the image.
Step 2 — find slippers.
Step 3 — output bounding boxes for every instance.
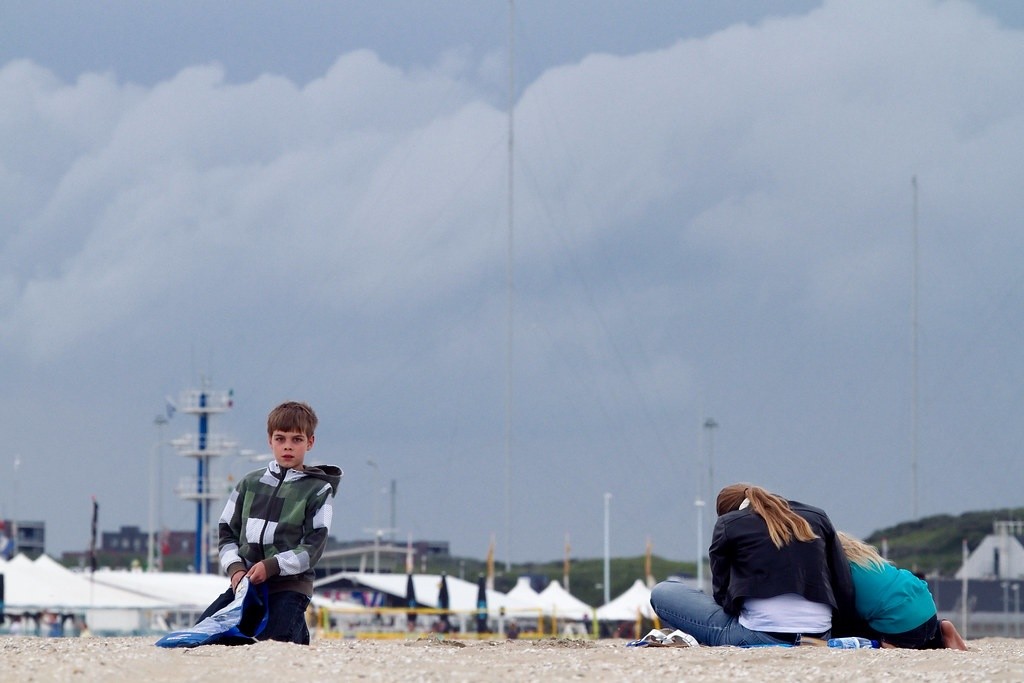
[640,628,676,646]
[664,629,708,649]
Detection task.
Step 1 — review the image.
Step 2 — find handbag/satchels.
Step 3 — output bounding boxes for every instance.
[154,576,270,648]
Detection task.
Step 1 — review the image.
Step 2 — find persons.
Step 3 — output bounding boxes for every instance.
[835,531,967,650]
[649,483,855,647]
[195,402,343,645]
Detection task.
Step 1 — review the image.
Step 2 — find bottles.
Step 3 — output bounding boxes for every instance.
[826,637,879,649]
